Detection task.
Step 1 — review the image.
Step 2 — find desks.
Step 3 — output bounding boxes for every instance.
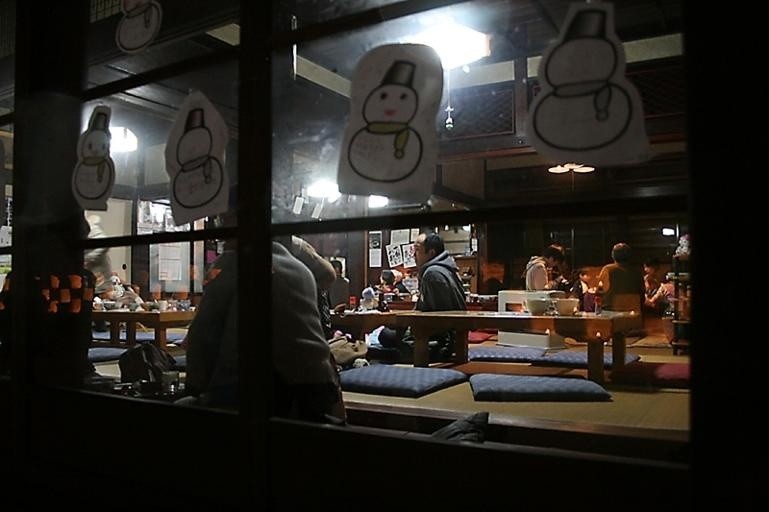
[90,307,196,353]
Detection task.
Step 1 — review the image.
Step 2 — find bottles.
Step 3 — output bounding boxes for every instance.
[349,295,356,311]
[593,296,602,315]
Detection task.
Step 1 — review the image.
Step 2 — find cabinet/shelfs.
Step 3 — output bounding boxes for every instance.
[664,250,690,358]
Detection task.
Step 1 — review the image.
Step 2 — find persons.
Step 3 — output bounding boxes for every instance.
[525,241,685,338]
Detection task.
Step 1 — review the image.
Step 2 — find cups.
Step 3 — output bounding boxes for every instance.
[92,300,192,312]
[551,299,580,317]
[522,297,552,316]
